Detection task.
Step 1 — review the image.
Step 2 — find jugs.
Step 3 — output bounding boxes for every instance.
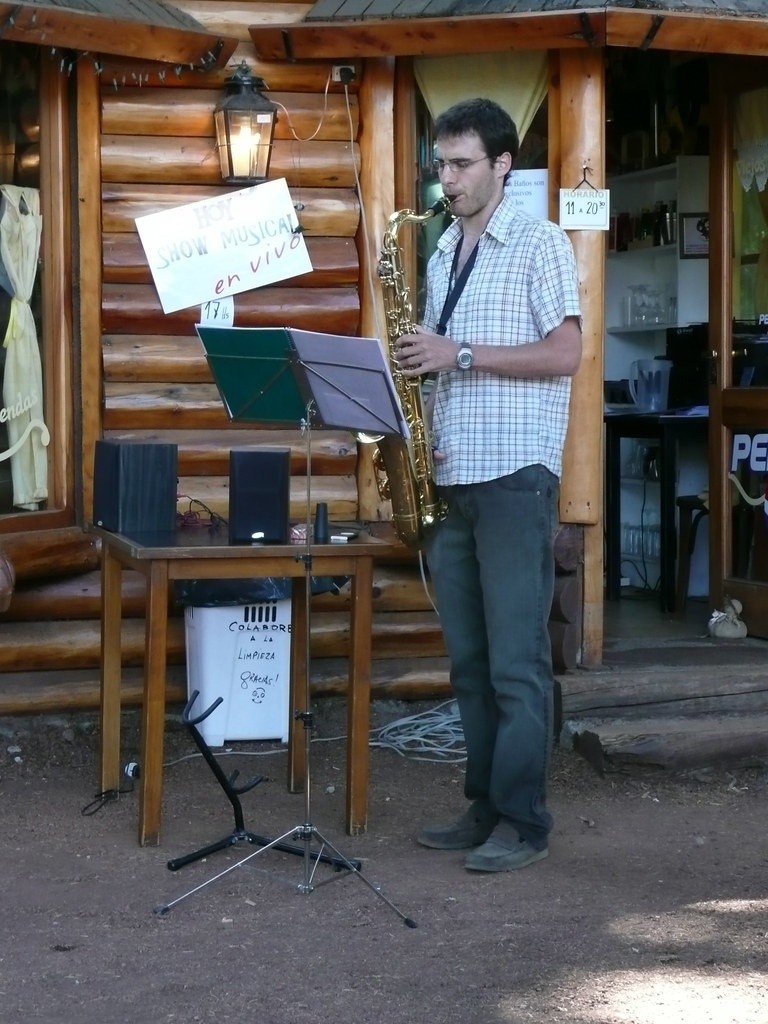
[629,359,673,412]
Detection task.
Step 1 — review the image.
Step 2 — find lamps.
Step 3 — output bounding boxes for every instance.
[215,58,279,186]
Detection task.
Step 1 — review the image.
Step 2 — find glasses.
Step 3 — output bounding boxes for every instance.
[433,156,496,172]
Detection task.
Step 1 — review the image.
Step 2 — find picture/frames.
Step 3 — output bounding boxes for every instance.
[676,211,712,259]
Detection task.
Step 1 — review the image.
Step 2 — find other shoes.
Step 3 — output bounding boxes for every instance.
[416,806,498,850]
[465,824,547,871]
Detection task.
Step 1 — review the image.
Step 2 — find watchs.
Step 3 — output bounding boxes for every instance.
[456,342,474,371]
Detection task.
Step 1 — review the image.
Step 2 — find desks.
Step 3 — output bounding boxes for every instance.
[597,413,710,617]
[87,527,395,848]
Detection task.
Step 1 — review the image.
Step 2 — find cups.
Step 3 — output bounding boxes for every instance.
[667,297,678,324]
[621,525,660,557]
[622,295,637,328]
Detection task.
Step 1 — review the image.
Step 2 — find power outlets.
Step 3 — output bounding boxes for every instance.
[332,64,356,83]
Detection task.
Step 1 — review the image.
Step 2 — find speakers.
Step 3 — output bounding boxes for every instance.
[229,446,291,546]
[92,439,179,533]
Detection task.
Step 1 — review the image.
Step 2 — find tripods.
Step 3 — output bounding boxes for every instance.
[161,323,418,928]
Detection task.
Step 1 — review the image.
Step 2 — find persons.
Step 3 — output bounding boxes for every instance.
[393,97,583,875]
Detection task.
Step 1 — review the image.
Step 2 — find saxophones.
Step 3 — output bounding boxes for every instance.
[356,190,458,548]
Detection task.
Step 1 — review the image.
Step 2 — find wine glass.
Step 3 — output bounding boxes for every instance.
[649,290,665,325]
[636,285,651,325]
